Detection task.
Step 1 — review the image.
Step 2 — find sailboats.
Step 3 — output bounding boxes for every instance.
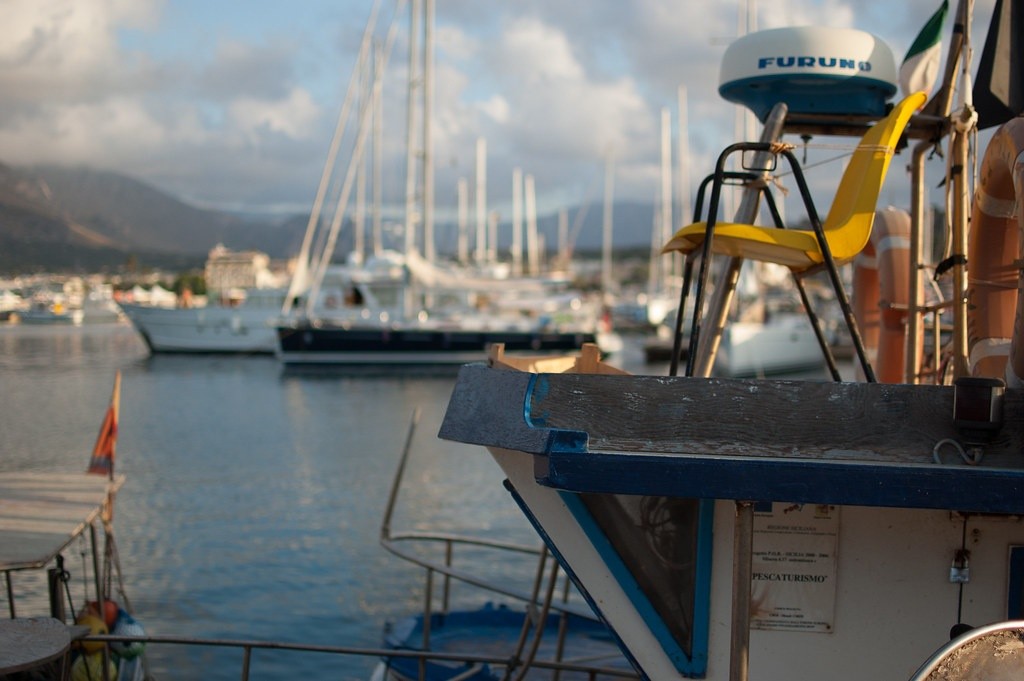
[1,0,1023,681]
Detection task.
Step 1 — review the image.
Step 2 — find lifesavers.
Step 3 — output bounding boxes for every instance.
[965,120,1024,390]
[853,205,912,386]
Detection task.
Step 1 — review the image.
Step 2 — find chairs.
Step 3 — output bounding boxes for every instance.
[663,90,926,383]
